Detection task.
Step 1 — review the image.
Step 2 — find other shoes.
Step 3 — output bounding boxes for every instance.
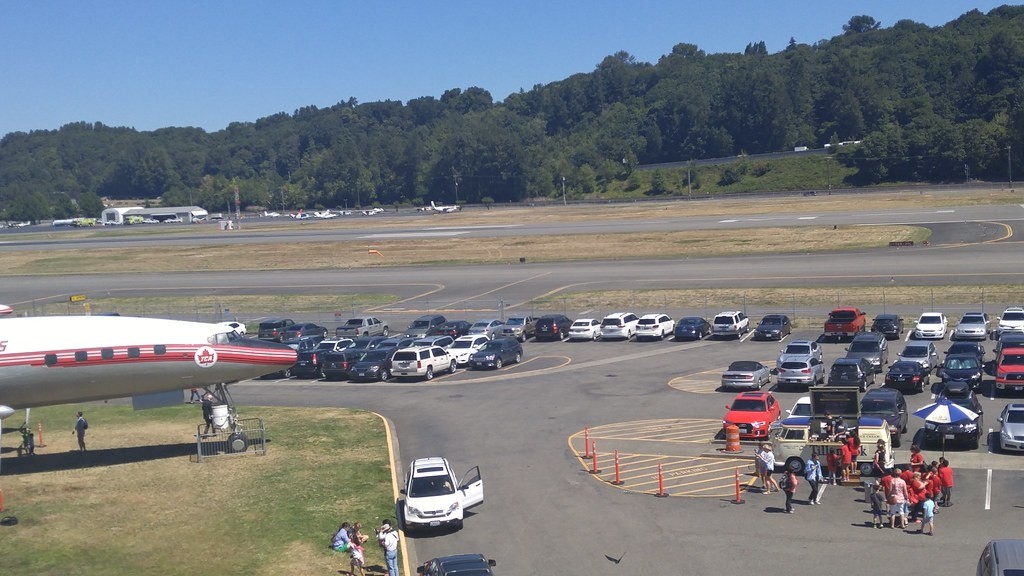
[851,470,857,474]
[840,476,846,481]
[869,507,939,536]
[845,477,849,482]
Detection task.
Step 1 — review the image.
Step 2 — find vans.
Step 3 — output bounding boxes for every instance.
[979,538,1024,576]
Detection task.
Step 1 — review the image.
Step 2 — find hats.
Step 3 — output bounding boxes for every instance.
[382,524,392,532]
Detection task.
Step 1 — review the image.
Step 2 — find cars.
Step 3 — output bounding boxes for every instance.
[993,333,1024,360]
[895,340,940,373]
[258,313,538,384]
[776,339,823,371]
[996,403,1024,453]
[722,392,781,441]
[218,321,248,339]
[721,360,773,391]
[995,347,1024,396]
[870,314,905,340]
[534,310,794,343]
[400,456,485,533]
[996,307,1024,336]
[914,312,949,340]
[785,396,812,419]
[954,312,993,340]
[423,553,497,576]
[941,343,986,390]
[884,360,931,396]
[826,357,876,393]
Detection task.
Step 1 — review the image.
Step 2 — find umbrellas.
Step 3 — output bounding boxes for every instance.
[913,400,979,457]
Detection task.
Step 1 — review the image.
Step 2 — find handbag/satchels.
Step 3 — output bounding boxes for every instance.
[331,536,336,543]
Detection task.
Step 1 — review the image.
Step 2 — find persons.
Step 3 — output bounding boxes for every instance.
[755,443,780,495]
[378,519,399,576]
[783,467,797,514]
[805,453,824,505]
[72,412,87,451]
[20,428,35,456]
[331,522,369,552]
[346,536,365,576]
[202,395,216,439]
[839,430,860,480]
[877,446,952,535]
[190,389,203,404]
[438,478,451,491]
[823,410,847,440]
[827,449,839,485]
[874,440,885,492]
[870,485,885,529]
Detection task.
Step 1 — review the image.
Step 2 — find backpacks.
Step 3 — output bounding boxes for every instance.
[777,473,792,489]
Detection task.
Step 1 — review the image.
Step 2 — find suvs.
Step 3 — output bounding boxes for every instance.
[859,387,909,449]
[925,380,984,450]
[844,331,889,374]
[776,356,826,392]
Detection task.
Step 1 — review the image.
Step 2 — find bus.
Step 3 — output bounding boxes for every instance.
[769,386,895,477]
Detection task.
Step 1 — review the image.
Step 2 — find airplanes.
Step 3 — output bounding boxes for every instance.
[258,200,462,220]
[0,314,299,451]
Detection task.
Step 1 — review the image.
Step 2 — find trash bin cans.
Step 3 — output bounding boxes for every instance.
[211,405,229,428]
[864,481,873,503]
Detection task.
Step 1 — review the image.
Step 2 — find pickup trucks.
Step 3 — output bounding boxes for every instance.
[824,308,867,342]
